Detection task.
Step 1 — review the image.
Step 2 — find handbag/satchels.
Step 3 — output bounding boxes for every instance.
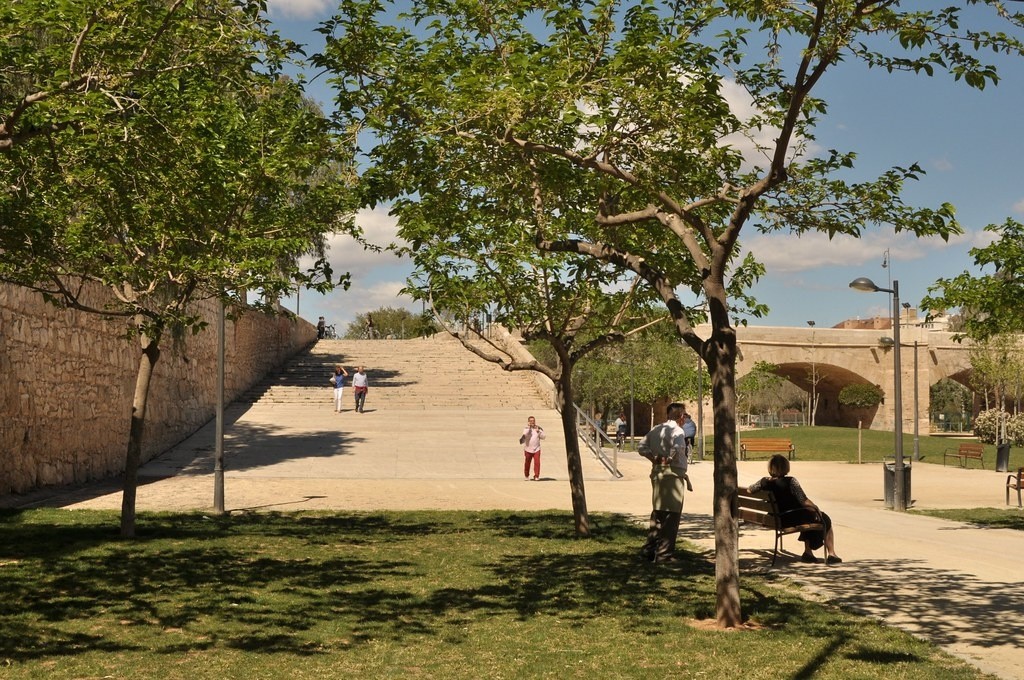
[329,373,336,384]
[520,434,526,444]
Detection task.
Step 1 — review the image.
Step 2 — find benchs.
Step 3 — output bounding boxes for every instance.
[944,443,984,469]
[1006,467,1024,507]
[736,493,826,566]
[738,438,794,460]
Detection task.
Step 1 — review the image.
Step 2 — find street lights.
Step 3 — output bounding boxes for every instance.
[615,359,635,450]
[577,370,593,439]
[848,276,906,512]
[682,340,703,461]
[879,337,919,461]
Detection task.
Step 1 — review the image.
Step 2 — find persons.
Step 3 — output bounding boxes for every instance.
[333,365,348,412]
[637,403,693,563]
[523,416,545,480]
[682,414,696,458]
[367,315,374,339]
[317,316,325,339]
[594,412,627,446]
[352,366,368,413]
[747,455,841,563]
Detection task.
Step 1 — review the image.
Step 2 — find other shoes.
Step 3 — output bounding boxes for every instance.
[525,477,529,481]
[355,409,358,412]
[335,410,337,412]
[534,477,540,481]
[827,555,842,564]
[359,408,364,413]
[802,553,818,562]
[338,410,341,413]
[617,443,620,447]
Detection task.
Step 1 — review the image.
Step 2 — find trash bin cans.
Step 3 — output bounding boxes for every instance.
[883,455,912,508]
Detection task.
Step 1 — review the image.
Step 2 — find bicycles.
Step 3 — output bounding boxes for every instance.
[325,324,339,340]
